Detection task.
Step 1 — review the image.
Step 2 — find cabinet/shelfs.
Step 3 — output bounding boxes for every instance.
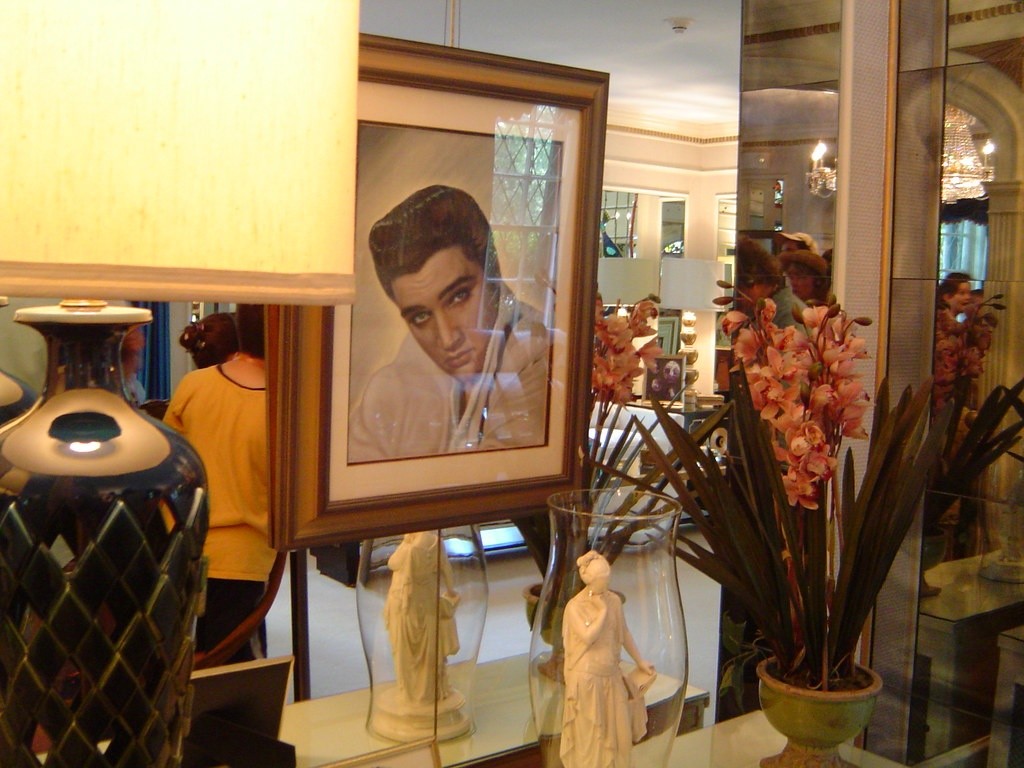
[633,708,905,768]
[279,651,708,768]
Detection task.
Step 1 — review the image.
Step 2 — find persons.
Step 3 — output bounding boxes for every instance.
[383,530,461,706]
[734,232,833,336]
[935,270,985,319]
[160,303,279,664]
[179,312,238,370]
[350,184,550,465]
[560,550,654,768]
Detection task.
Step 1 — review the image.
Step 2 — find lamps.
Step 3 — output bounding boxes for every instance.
[659,257,725,403]
[941,104,994,204]
[0,2,360,768]
[805,141,838,198]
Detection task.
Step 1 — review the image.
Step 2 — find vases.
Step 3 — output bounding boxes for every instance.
[527,489,689,768]
[757,655,883,768]
[525,584,626,684]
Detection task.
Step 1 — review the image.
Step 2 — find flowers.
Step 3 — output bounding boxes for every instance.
[509,271,1024,689]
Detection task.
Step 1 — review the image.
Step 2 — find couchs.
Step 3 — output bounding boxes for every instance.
[588,400,687,544]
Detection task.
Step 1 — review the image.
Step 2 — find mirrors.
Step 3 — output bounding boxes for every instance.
[660,201,685,254]
[599,190,639,259]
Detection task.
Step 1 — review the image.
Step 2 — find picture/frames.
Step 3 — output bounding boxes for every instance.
[642,354,686,406]
[265,33,610,551]
[603,305,683,354]
[178,655,297,768]
